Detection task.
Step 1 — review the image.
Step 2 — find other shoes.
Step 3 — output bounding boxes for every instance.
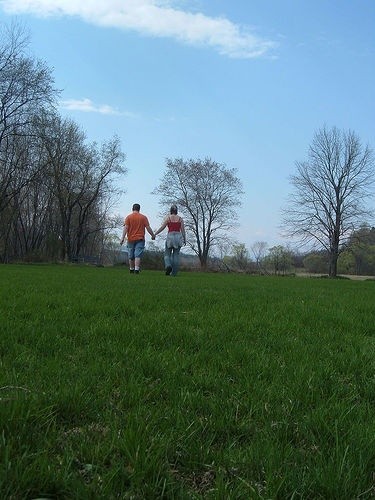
[135,270,139,273]
[130,270,134,273]
[165,265,172,275]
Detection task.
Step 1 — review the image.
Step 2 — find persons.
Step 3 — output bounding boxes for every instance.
[151,205,186,276]
[120,204,154,274]
